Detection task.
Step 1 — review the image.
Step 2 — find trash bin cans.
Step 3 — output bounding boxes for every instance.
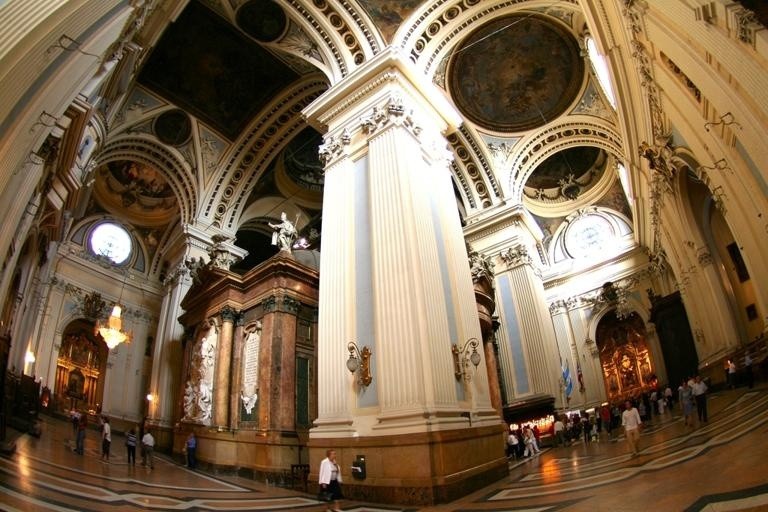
[291,463,310,493]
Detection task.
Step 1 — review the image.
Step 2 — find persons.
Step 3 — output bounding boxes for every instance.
[268,211,294,252]
[186,432,197,468]
[184,255,205,287]
[507,350,759,458]
[100,417,112,460]
[72,411,79,433]
[141,429,156,469]
[73,409,88,455]
[318,449,343,512]
[127,428,137,464]
[653,139,678,178]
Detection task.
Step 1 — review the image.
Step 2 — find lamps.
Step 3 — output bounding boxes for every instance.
[347,342,372,386]
[94,267,133,349]
[452,338,481,384]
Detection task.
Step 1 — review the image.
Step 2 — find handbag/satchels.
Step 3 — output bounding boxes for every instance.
[317,488,334,502]
